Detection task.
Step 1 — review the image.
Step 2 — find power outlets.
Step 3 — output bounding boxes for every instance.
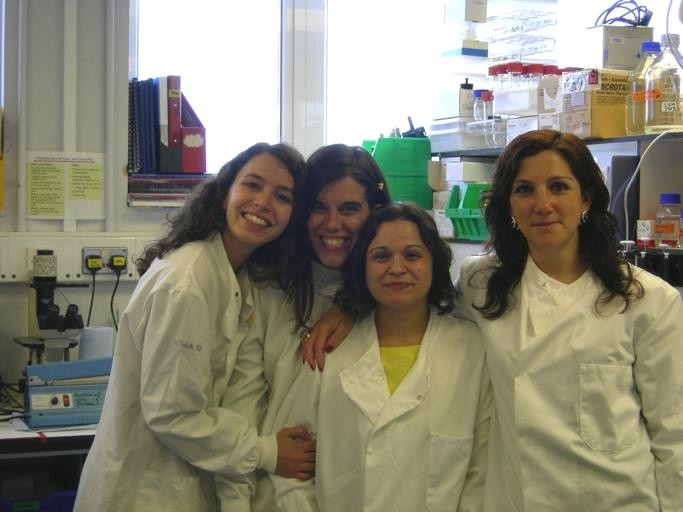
[82,246,127,276]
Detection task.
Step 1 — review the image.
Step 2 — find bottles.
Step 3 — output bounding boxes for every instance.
[466,90,508,119]
[489,61,579,112]
[481,116,506,147]
[655,193,681,247]
[625,33,683,134]
[459,78,475,119]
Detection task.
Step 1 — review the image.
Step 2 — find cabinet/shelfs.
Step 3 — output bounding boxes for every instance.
[427,132,683,296]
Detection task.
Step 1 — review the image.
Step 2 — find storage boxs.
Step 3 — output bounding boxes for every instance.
[428,161,493,240]
[539,69,632,142]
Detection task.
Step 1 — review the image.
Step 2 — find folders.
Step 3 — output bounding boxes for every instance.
[156,76,180,146]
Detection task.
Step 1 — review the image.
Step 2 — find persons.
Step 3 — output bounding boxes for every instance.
[74,128,495,512]
[302,130,682,511]
